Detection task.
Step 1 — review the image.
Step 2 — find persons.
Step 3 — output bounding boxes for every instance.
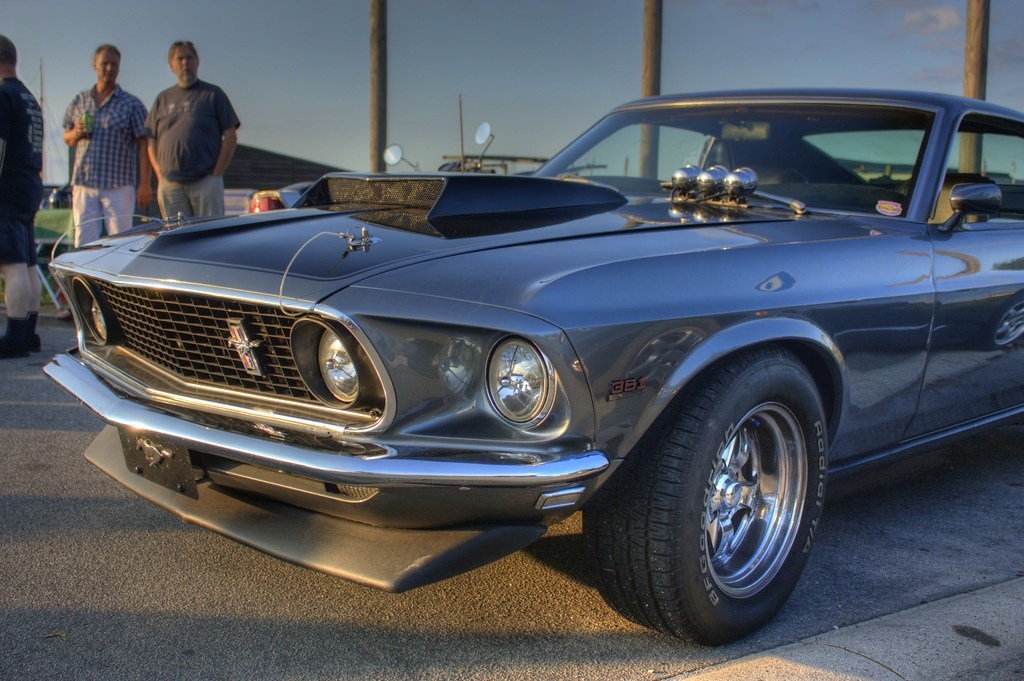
[144,39,241,222]
[62,43,149,250]
[0,34,46,356]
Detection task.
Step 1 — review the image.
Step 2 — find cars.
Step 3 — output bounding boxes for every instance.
[41,82,1023,644]
[33,187,76,318]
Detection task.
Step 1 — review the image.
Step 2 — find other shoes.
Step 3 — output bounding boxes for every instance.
[0,338,21,357]
[24,335,40,351]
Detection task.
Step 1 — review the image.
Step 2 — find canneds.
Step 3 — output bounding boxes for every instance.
[82,113,93,132]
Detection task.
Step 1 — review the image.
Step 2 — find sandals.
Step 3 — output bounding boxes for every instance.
[57,307,72,321]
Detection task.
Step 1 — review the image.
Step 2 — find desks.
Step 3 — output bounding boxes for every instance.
[34,239,72,310]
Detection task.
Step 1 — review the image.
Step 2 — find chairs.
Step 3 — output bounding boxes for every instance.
[897,172,996,224]
[746,165,807,185]
